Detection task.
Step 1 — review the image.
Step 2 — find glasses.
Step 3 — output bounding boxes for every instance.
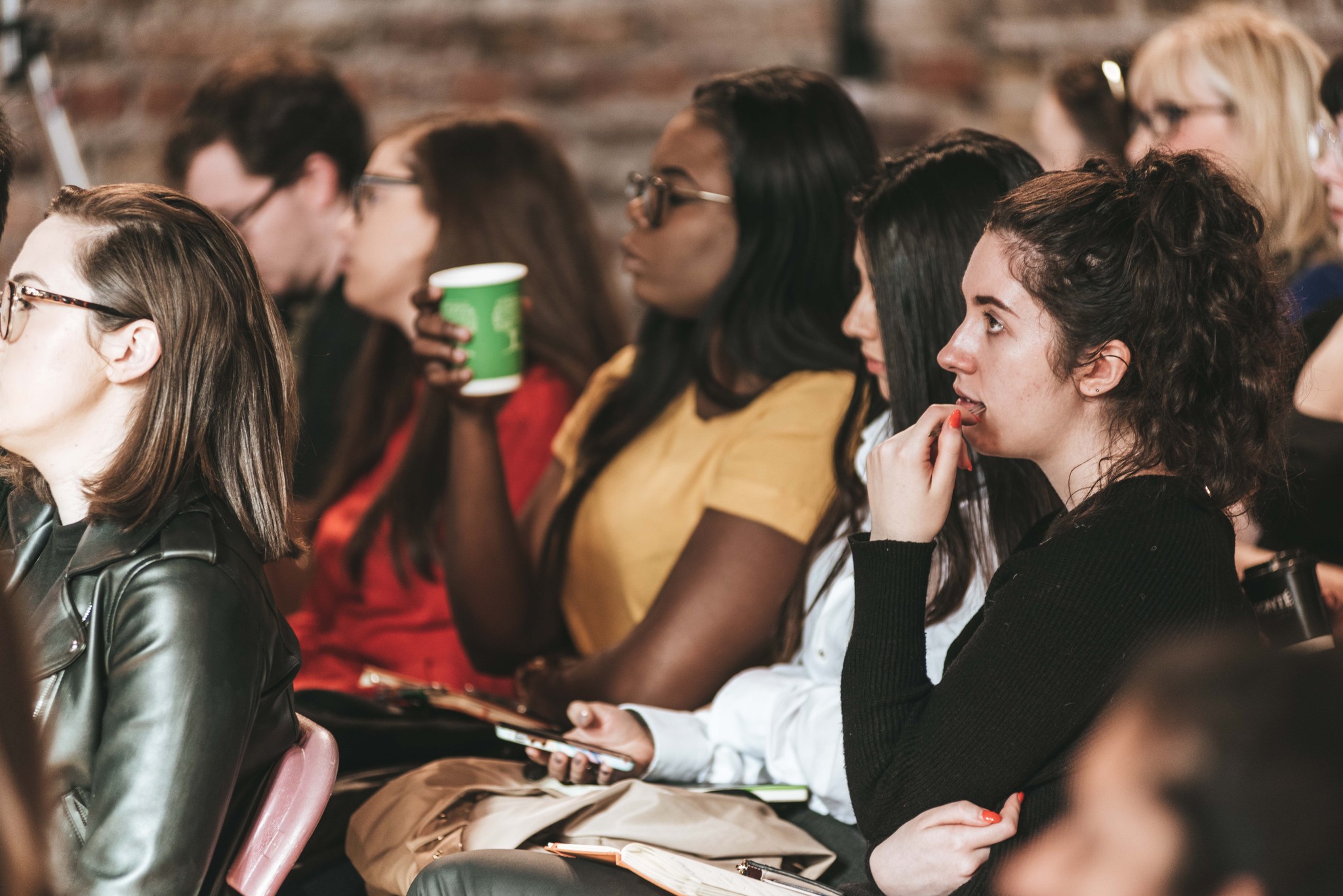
[0,279,134,341]
[624,170,732,229]
[1308,123,1341,179]
[350,172,421,224]
[1125,99,1229,145]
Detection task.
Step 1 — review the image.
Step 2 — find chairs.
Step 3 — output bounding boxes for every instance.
[222,712,341,895]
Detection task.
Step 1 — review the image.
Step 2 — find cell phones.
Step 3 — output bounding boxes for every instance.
[494,722,635,772]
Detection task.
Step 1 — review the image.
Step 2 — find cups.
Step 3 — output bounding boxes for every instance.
[1242,553,1331,652]
[430,262,528,396]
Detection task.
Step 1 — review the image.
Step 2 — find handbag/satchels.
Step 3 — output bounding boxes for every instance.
[290,685,556,777]
[345,758,838,896]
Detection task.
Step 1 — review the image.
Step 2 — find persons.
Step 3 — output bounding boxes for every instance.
[159,9,1343,896]
[0,182,306,896]
